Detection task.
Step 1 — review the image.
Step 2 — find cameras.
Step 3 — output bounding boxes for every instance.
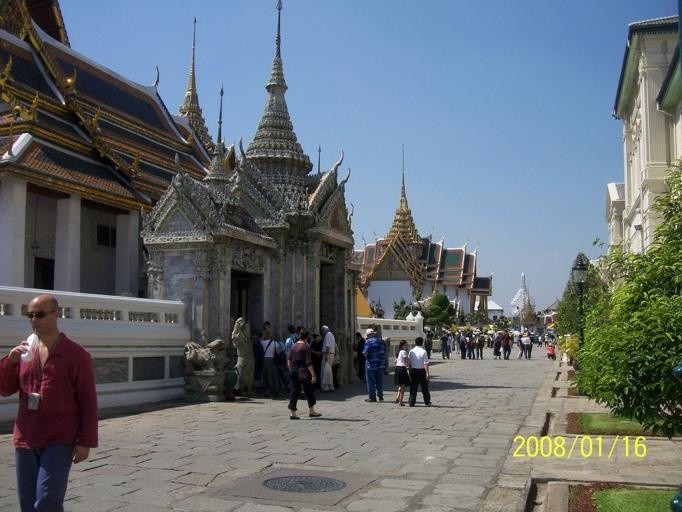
[27,392,40,411]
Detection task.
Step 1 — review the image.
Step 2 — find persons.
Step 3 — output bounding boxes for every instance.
[251,322,433,422]
[1,294,98,512]
[441,325,560,361]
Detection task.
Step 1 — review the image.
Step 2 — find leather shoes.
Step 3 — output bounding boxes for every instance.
[394,398,432,407]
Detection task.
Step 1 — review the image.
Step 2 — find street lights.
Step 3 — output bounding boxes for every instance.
[571,255,588,395]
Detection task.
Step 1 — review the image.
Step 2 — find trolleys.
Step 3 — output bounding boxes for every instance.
[544,341,558,362]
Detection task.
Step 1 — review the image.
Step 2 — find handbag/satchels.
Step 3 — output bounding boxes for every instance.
[273,351,286,366]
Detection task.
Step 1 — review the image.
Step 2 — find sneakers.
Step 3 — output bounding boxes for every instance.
[309,414,322,417]
[290,416,300,419]
[379,397,383,400]
[365,398,377,402]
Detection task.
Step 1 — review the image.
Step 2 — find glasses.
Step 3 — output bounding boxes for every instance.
[26,312,55,319]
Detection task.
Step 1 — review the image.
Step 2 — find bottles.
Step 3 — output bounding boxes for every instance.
[21,334,40,363]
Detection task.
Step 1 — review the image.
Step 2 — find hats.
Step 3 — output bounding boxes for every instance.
[362,328,377,339]
